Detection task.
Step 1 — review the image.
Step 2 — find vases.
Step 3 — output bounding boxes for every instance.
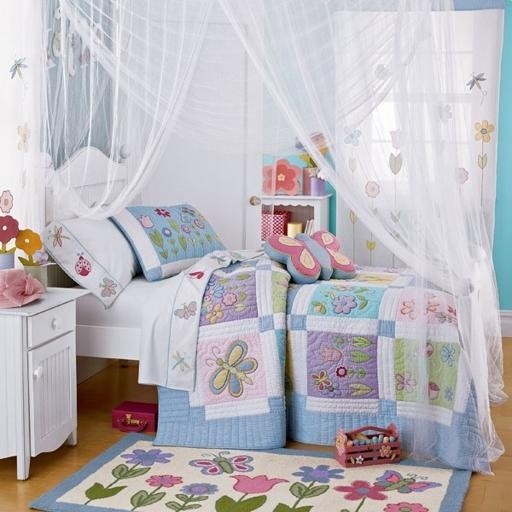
[0,253,14,270]
[303,166,326,196]
[24,265,47,293]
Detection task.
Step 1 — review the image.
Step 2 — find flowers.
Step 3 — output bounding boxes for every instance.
[15,228,43,266]
[0,215,20,255]
[295,134,328,168]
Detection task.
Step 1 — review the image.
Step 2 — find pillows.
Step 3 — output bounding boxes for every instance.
[265,231,358,285]
[39,198,140,310]
[100,203,228,282]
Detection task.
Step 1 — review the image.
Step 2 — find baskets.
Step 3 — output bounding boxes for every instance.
[262,210,289,240]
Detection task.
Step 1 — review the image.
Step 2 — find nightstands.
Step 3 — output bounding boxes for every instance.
[0,286,93,481]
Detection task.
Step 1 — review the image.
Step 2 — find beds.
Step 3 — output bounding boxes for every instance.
[44,145,481,387]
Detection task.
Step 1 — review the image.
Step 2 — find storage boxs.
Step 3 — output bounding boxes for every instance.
[111,400,159,432]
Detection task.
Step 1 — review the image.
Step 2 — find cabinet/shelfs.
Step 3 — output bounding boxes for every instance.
[244,192,334,250]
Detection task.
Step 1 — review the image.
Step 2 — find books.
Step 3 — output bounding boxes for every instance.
[304,220,315,237]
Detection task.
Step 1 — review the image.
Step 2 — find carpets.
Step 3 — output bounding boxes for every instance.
[27,433,473,512]
